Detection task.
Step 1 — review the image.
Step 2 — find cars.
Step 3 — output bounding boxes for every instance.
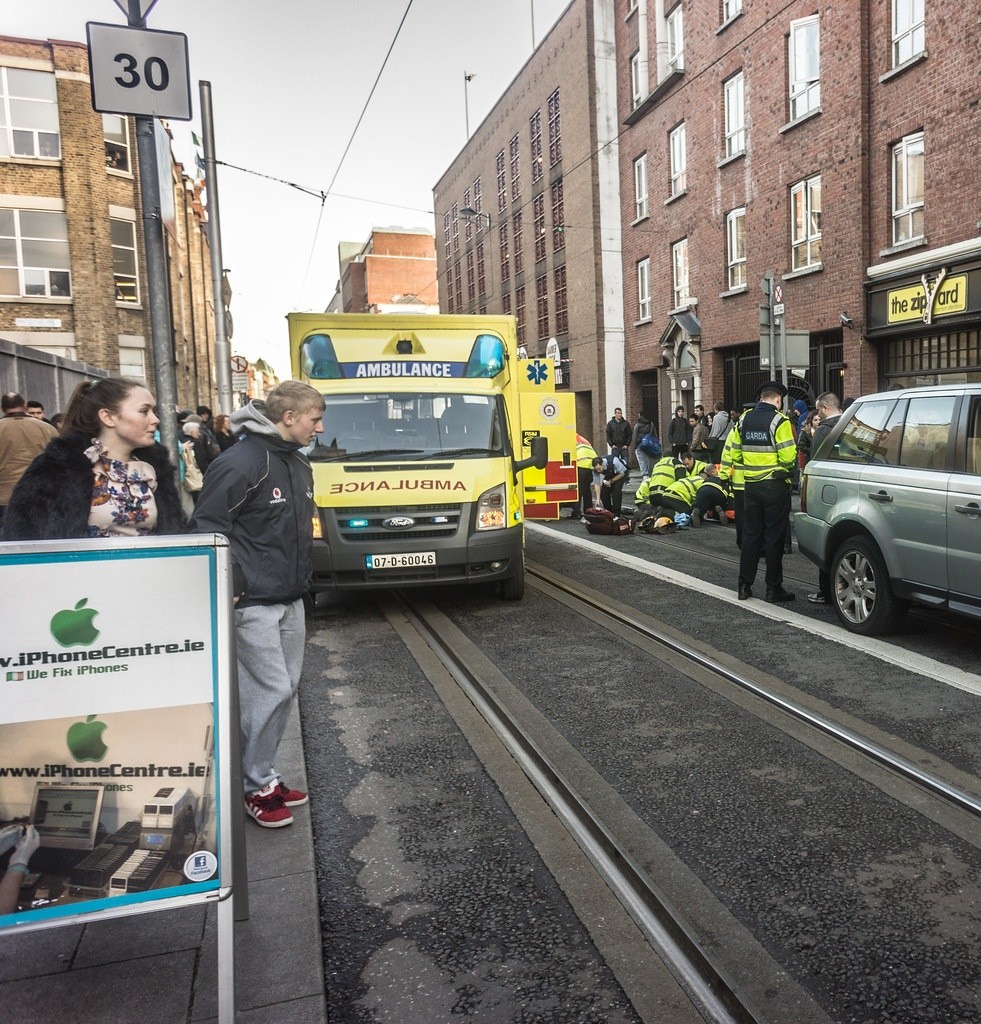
[792,385,981,633]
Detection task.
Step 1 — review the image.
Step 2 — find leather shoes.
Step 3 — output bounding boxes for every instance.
[766,588,795,602]
[738,585,753,600]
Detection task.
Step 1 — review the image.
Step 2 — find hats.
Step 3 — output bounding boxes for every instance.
[181,414,202,423]
[196,406,212,416]
[757,381,788,410]
[743,403,756,407]
[176,410,192,419]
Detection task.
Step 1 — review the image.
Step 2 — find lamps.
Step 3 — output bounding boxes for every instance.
[460,208,490,227]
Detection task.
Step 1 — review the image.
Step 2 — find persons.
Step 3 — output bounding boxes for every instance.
[798,388,855,605]
[0,377,188,542]
[0,393,70,510]
[569,401,744,533]
[194,380,326,827]
[719,382,800,602]
[148,404,236,528]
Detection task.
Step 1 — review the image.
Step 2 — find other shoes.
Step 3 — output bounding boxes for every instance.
[566,515,582,519]
[715,505,729,525]
[625,482,630,486]
[691,508,701,528]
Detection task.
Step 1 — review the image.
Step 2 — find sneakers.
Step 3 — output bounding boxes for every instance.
[269,778,309,807]
[808,593,825,603]
[245,786,294,827]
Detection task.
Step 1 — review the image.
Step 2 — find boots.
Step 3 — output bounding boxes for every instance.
[634,504,675,522]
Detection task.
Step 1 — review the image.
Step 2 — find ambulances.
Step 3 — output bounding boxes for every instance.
[282,309,578,601]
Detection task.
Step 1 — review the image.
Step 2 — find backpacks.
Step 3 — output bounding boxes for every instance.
[582,507,618,536]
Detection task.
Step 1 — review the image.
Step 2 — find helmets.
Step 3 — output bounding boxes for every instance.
[638,516,680,533]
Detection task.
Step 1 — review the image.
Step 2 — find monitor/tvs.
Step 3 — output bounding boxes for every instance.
[28,785,104,872]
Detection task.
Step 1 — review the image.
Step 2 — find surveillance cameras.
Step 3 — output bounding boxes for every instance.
[840,313,852,325]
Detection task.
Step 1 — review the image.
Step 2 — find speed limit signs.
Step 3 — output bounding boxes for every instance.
[85,22,191,123]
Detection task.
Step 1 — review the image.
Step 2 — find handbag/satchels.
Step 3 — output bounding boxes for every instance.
[182,440,204,492]
[639,421,662,459]
[704,437,720,451]
